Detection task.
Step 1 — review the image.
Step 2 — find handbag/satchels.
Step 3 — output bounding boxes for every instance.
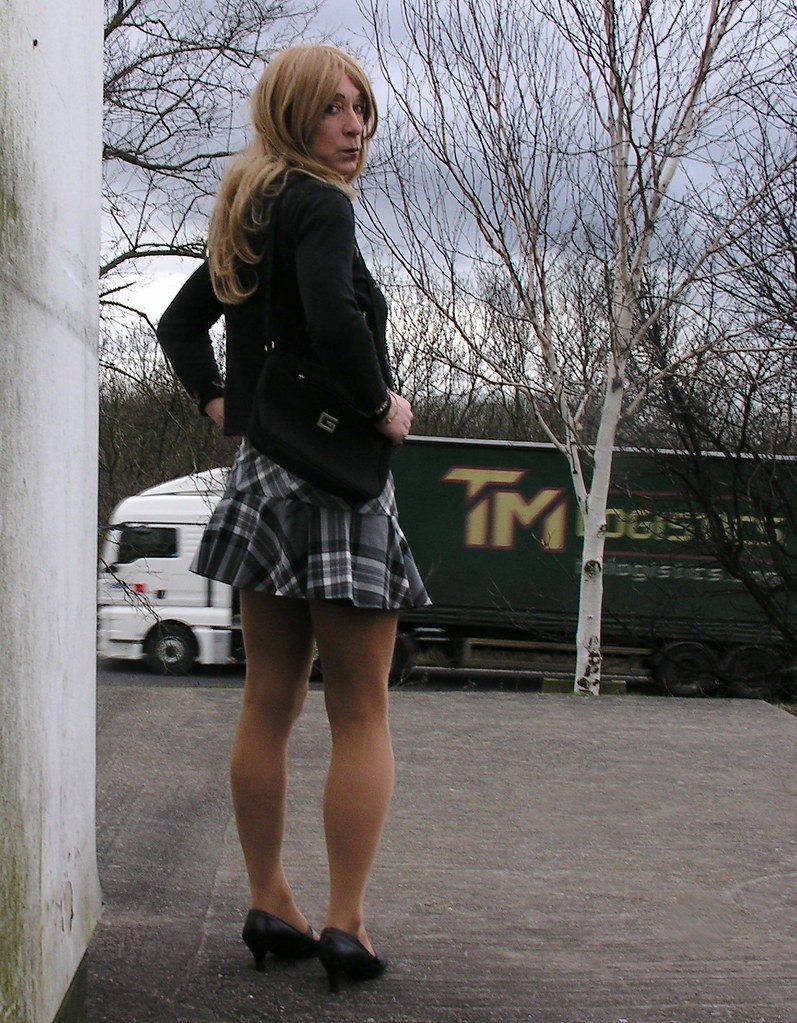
[246,174,393,509]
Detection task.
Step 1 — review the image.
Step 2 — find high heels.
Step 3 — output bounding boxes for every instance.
[241,908,320,972]
[315,927,388,993]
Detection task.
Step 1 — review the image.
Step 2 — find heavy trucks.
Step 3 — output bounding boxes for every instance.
[97,433,797,703]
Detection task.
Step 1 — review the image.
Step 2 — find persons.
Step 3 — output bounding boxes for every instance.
[155,47,434,1002]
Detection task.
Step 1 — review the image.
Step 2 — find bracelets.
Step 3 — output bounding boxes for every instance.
[386,391,399,426]
